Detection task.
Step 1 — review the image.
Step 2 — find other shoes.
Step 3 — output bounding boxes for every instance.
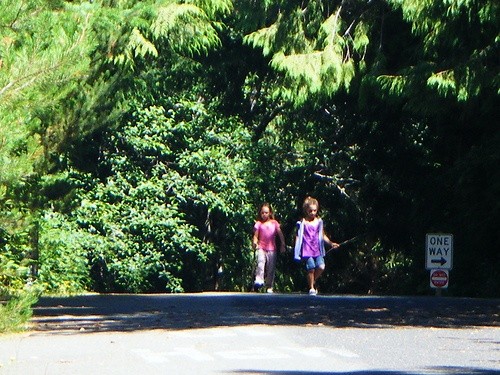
[267,288,273,293]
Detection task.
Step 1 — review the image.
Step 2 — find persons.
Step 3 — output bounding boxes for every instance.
[294,196,342,295]
[252,203,287,294]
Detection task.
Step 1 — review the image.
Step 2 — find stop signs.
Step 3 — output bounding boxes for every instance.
[431,270,448,287]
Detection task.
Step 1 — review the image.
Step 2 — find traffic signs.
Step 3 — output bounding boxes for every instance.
[425,233,454,290]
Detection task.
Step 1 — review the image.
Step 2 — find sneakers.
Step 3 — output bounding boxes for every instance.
[309,289,317,296]
